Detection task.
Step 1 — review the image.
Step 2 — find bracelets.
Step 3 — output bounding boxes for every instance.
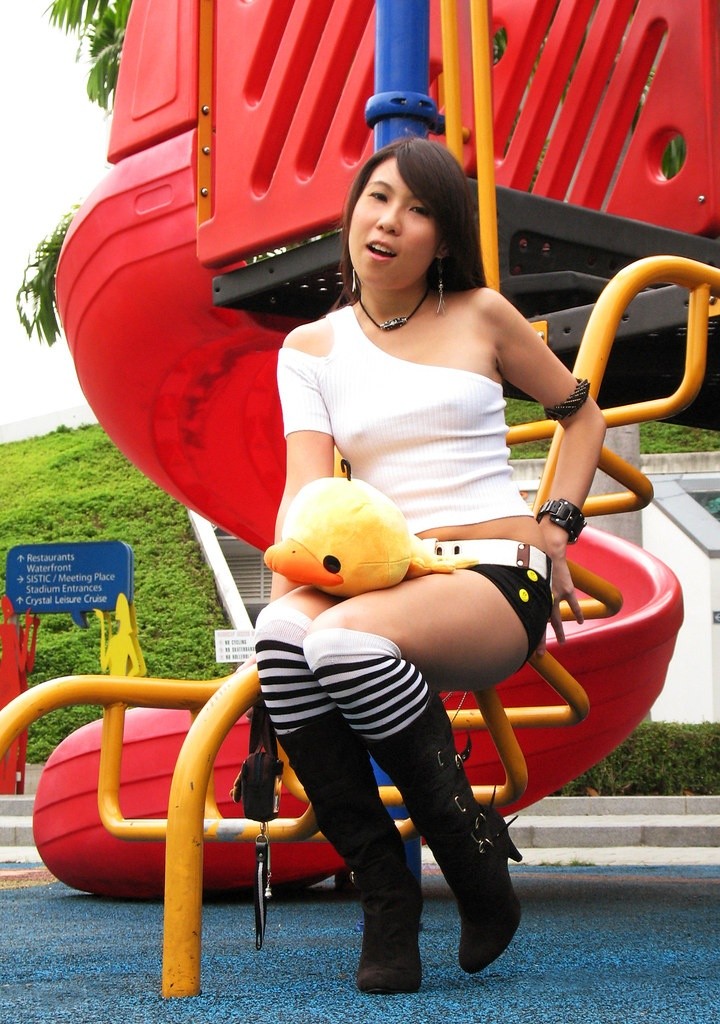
[535,498,588,545]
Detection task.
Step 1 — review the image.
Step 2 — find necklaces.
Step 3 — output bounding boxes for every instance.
[357,284,432,331]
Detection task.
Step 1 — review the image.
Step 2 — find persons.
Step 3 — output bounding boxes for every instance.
[254,134,609,994]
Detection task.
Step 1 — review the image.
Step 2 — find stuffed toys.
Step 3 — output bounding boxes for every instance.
[263,458,480,599]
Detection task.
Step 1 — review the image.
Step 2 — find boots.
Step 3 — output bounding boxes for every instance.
[363,689,523,973]
[276,705,425,994]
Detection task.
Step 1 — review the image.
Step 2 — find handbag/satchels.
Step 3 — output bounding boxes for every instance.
[229,698,284,948]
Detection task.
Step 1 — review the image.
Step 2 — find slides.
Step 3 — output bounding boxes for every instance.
[33,126,685,898]
